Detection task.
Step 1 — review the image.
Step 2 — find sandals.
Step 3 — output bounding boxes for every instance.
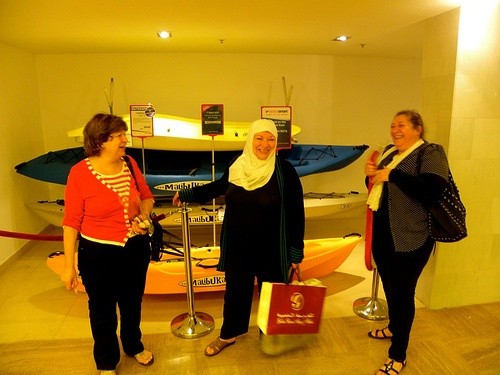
[367,326,392,340]
[375,357,407,375]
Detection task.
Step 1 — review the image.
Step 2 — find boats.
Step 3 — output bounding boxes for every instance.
[12,141,371,200]
[46,231,364,295]
[65,112,302,150]
[25,191,366,230]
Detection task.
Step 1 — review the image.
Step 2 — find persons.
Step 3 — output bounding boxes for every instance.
[173,118,306,356]
[364,110,448,375]
[61,114,154,375]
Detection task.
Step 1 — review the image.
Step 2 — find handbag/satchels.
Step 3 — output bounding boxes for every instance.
[122,153,165,262]
[413,143,468,243]
[256,265,327,336]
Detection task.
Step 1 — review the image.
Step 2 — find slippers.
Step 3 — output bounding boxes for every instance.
[134,350,155,366]
[204,337,236,356]
